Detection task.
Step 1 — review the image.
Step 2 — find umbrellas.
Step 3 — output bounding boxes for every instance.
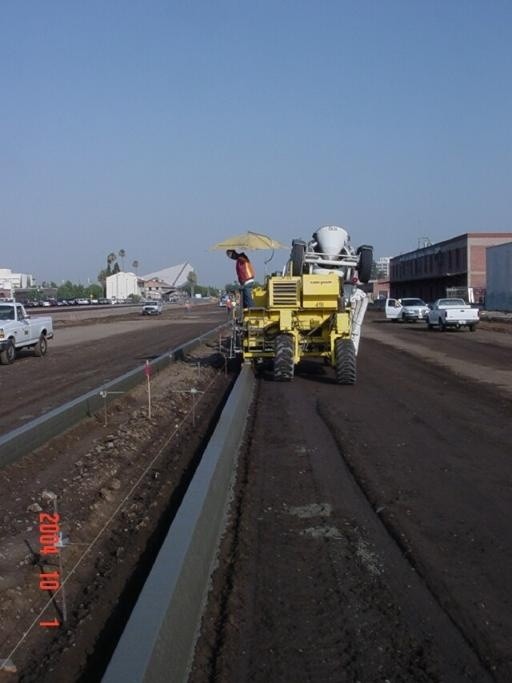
[213,231,288,262]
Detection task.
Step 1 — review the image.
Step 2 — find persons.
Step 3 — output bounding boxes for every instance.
[227,250,256,308]
[226,294,241,322]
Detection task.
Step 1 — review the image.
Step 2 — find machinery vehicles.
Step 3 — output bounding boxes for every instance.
[234,226,373,386]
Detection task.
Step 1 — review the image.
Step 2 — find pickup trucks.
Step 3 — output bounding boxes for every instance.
[142,301,163,315]
[0,302,53,364]
[427,299,481,331]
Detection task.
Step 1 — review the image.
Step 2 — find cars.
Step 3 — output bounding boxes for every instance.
[385,296,430,322]
[220,295,232,307]
[26,299,124,306]
